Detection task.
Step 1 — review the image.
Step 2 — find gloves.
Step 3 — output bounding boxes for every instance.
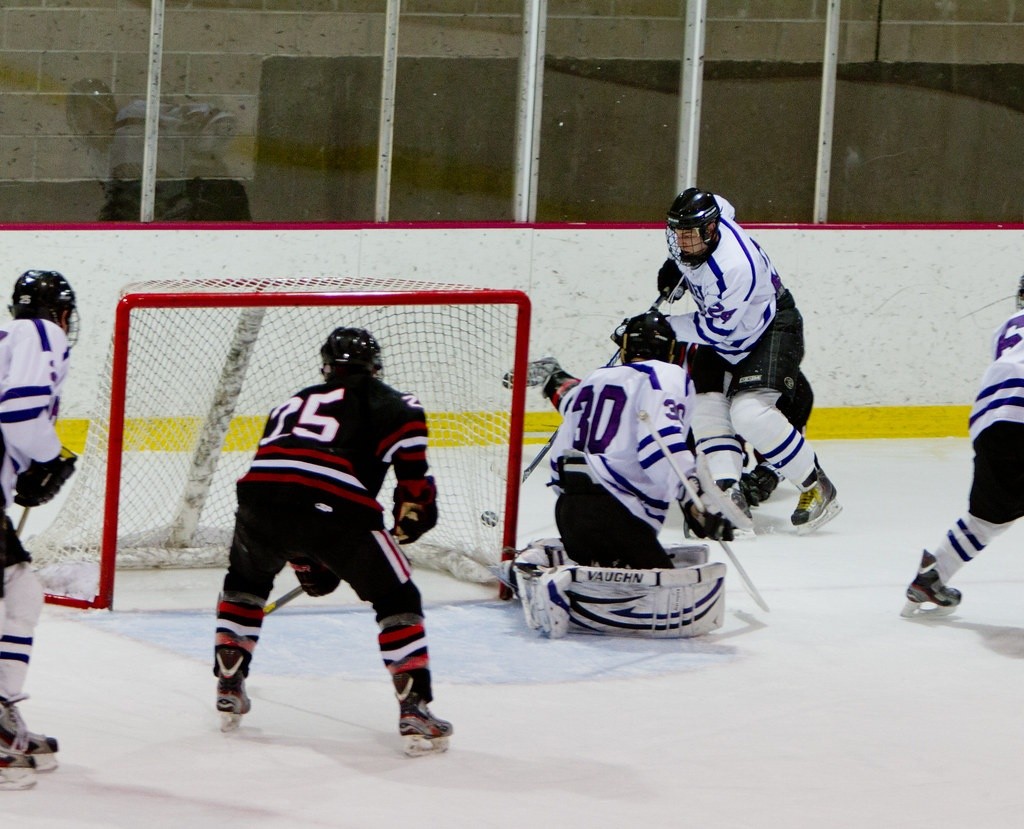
[658,257,688,304]
[676,476,734,541]
[14,460,53,507]
[390,476,437,545]
[288,556,340,597]
[610,318,630,347]
[39,447,78,495]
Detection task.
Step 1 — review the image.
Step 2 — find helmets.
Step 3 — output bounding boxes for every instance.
[321,327,383,381]
[666,188,721,264]
[65,78,118,154]
[7,269,79,348]
[620,311,677,365]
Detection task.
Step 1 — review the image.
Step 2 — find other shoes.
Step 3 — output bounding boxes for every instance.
[510,538,554,594]
[513,564,571,640]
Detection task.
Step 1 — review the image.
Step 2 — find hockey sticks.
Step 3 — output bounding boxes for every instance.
[488,293,666,488]
[636,408,773,616]
[214,526,398,622]
[14,504,32,541]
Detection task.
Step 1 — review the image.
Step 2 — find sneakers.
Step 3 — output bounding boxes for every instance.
[214,648,251,732]
[791,452,842,536]
[392,667,453,757]
[0,693,59,772]
[0,739,38,791]
[503,356,563,399]
[900,548,961,618]
[689,488,755,540]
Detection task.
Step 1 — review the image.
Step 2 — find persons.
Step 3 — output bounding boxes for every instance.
[65,77,253,222]
[486,186,842,642]
[0,268,83,791]
[212,326,455,760]
[900,272,1024,622]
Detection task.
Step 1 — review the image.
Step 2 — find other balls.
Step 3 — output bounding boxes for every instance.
[480,510,499,529]
